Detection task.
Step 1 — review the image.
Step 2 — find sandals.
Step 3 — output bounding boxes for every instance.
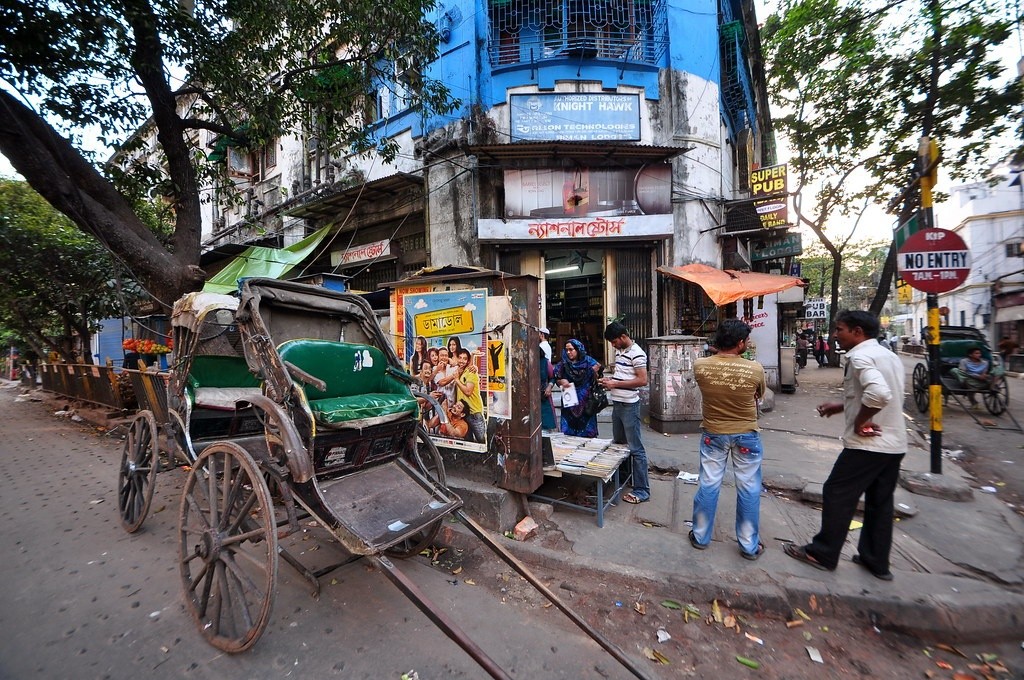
[689,529,707,549]
[740,542,765,561]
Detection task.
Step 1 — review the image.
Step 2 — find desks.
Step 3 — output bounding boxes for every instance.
[529,431,633,526]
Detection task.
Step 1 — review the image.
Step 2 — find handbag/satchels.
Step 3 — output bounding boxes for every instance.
[585,366,608,416]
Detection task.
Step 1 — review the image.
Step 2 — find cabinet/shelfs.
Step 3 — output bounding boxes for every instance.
[545,272,605,345]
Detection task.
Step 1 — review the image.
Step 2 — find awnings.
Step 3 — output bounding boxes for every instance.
[654,261,802,307]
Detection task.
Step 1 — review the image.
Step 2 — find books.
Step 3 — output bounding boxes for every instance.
[541,427,629,476]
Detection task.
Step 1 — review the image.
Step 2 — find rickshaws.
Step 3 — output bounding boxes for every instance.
[911,325,1023,437]
[117,273,644,680]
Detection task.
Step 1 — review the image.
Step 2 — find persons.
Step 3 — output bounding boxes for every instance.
[879,333,889,349]
[782,307,907,582]
[407,335,486,445]
[949,347,1006,393]
[997,334,1019,361]
[539,346,557,431]
[920,331,927,345]
[796,333,808,369]
[890,333,898,355]
[911,334,916,344]
[596,321,650,504]
[815,336,829,368]
[71,347,79,361]
[538,326,552,362]
[688,318,767,560]
[553,338,601,438]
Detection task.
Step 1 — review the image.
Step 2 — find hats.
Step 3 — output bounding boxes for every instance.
[539,328,550,334]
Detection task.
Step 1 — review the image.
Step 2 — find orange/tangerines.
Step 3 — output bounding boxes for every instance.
[122,338,172,354]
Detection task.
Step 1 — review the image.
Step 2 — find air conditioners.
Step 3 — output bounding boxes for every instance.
[394,51,418,76]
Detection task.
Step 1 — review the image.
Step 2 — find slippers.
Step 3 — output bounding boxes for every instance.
[852,554,896,579]
[783,540,830,570]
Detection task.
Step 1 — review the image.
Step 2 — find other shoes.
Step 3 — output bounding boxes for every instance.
[818,363,825,368]
[990,384,1001,393]
[622,493,642,503]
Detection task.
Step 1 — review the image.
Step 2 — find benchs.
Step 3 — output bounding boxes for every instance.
[938,339,994,368]
[276,335,419,428]
[177,352,264,410]
